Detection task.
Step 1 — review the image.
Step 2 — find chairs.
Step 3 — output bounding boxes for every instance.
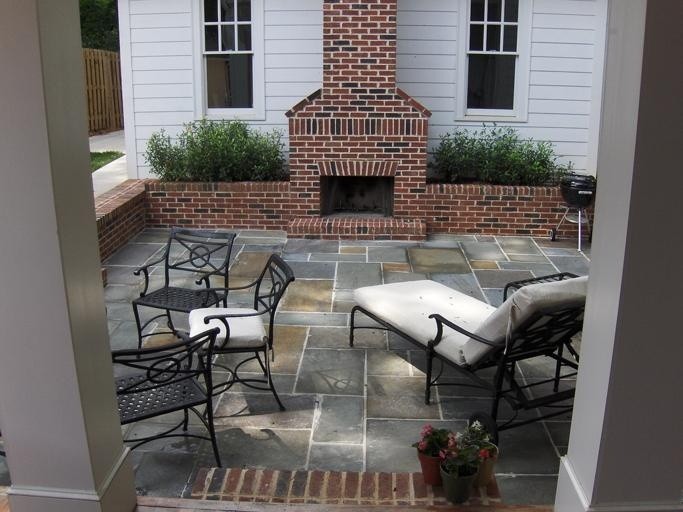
[132,226,236,372]
[350,277,588,428]
[188,253,294,420]
[111,328,222,468]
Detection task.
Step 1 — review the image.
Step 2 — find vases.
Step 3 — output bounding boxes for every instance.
[417,444,499,504]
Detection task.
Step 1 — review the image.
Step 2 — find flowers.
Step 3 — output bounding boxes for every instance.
[411,420,497,477]
